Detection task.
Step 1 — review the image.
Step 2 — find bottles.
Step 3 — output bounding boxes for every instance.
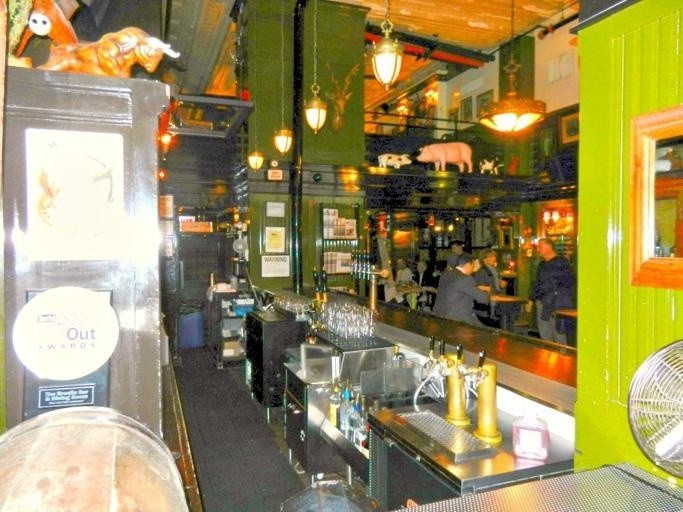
[351,249,370,268]
[328,387,367,448]
[312,265,328,292]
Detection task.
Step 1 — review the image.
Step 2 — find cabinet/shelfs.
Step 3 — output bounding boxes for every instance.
[207,290,260,370]
[245,309,309,408]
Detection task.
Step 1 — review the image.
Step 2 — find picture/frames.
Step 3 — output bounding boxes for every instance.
[260,254,291,279]
[265,200,286,219]
[264,226,286,254]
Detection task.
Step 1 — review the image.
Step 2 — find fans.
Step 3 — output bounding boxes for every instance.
[628,339,683,479]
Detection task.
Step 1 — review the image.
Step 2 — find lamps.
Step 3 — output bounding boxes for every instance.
[369,0,405,91]
[479,0,546,135]
[247,0,264,172]
[273,0,294,158]
[303,0,328,136]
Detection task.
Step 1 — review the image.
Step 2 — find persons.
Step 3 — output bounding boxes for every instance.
[390,238,508,329]
[528,238,574,346]
